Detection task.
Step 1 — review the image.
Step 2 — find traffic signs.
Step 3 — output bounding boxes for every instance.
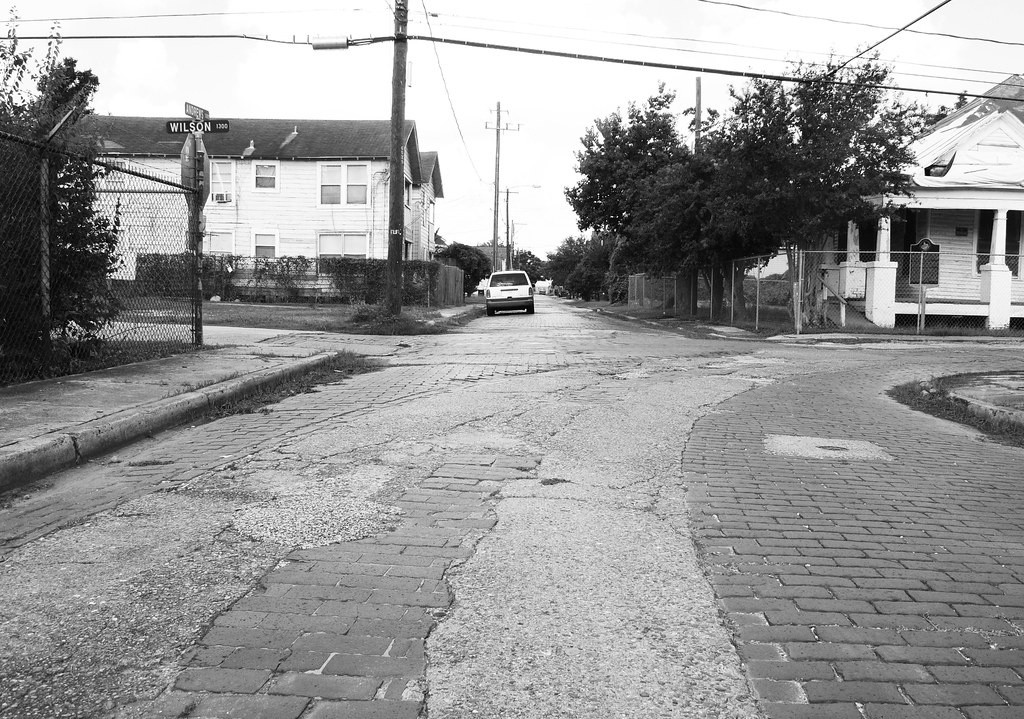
[166,120,230,134]
[185,102,210,121]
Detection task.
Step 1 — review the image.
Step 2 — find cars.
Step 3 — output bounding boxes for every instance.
[485,270,535,314]
